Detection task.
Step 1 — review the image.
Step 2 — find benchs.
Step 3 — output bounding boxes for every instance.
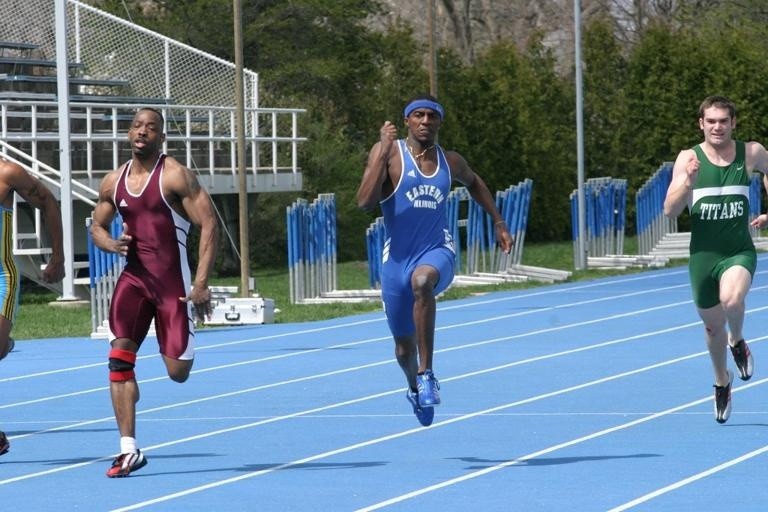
[0,41,219,134]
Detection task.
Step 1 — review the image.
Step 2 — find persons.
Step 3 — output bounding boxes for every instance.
[90,107,220,477]
[0,159,66,454]
[356,94,514,427]
[664,95,768,423]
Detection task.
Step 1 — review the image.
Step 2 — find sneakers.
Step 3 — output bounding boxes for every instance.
[406,387,434,426]
[108,449,147,478]
[416,370,441,406]
[1,433,8,453]
[729,337,752,380]
[715,370,733,423]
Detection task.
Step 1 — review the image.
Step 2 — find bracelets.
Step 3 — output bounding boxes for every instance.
[493,220,506,225]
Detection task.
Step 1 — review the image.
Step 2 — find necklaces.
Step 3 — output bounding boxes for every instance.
[405,138,435,159]
